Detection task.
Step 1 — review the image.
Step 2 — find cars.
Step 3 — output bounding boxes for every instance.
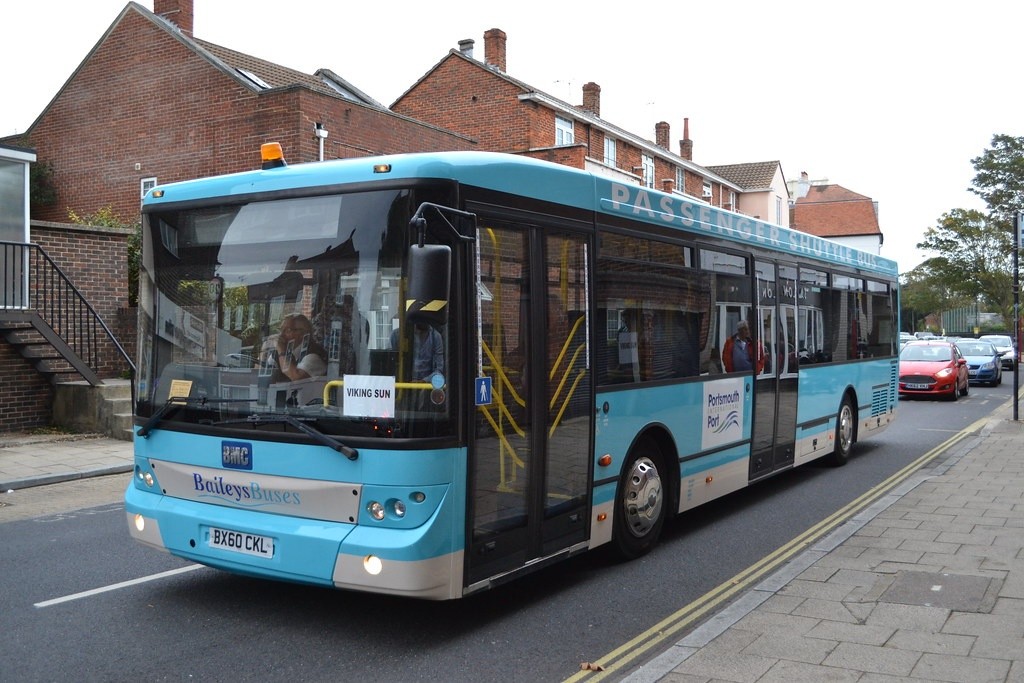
[954,342,1005,388]
[775,342,797,373]
[900,332,976,355]
[976,335,1015,372]
[899,341,969,402]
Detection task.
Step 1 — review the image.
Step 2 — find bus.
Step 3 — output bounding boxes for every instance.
[124,142,900,602]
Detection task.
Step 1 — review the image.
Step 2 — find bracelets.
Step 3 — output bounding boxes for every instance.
[278,352,288,357]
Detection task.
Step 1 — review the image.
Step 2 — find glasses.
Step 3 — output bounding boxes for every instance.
[276,327,304,335]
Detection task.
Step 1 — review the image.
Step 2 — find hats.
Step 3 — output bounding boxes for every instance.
[736,320,748,329]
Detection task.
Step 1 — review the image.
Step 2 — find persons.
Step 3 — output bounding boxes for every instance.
[509,294,652,422]
[722,320,766,373]
[253,293,443,407]
